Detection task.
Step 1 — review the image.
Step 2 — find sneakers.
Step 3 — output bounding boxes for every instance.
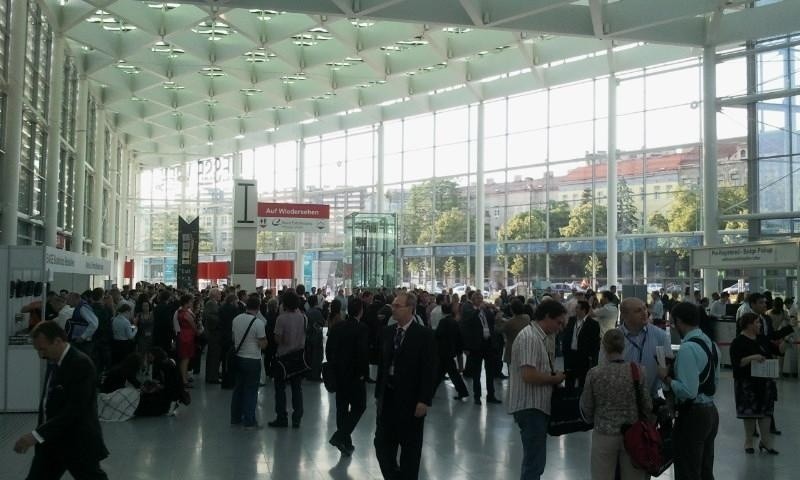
[486,396,502,404]
[268,417,289,427]
[185,368,222,389]
[292,413,300,427]
[454,391,469,400]
[498,373,510,379]
[474,398,482,405]
[769,429,781,436]
[230,418,264,431]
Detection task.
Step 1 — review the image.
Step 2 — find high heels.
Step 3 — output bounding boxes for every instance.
[744,443,755,453]
[758,441,779,456]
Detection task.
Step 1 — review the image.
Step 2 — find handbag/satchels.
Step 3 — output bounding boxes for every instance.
[653,396,680,477]
[547,386,594,438]
[271,348,313,384]
[221,356,235,390]
[621,423,661,471]
[65,319,95,343]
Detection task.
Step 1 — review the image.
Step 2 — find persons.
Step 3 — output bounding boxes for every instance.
[14,281,800,480]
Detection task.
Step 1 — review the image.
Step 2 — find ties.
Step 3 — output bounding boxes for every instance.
[394,328,403,351]
